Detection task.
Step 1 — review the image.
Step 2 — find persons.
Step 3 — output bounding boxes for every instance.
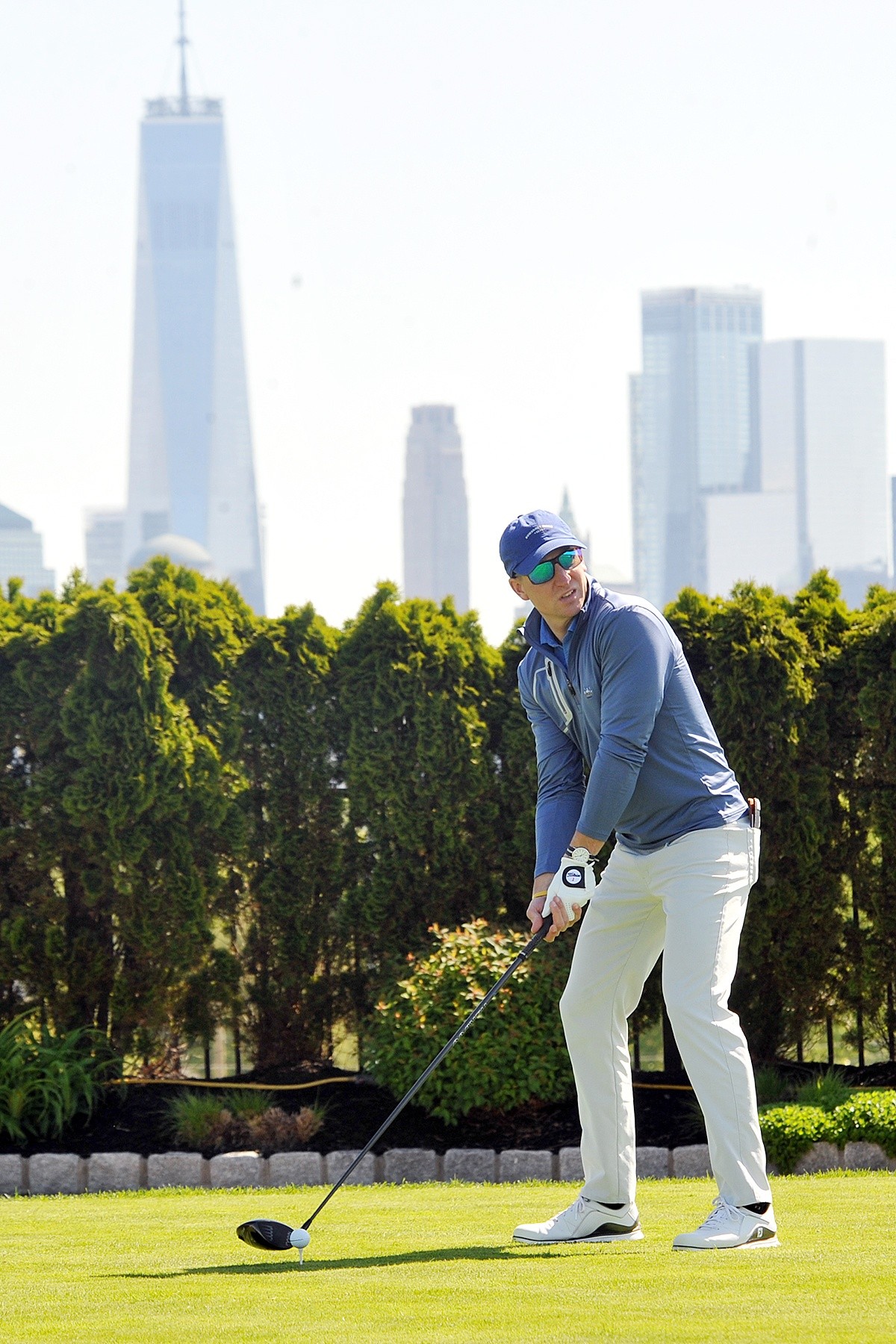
[498,511,778,1253]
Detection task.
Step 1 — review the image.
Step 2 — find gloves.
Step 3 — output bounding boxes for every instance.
[540,845,597,922]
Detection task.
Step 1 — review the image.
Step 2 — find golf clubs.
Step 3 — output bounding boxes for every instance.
[235,913,554,1251]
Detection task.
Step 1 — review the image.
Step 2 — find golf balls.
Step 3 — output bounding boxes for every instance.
[290,1229,310,1248]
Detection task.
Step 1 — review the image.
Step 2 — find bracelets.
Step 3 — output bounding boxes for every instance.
[532,890,547,899]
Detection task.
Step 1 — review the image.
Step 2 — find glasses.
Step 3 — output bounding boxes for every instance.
[528,547,583,586]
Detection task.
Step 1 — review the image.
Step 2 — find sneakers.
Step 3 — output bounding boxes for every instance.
[511,1193,643,1245]
[671,1201,778,1253]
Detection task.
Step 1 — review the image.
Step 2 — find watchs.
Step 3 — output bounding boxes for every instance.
[566,845,594,864]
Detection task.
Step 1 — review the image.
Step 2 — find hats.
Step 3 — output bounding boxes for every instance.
[497,510,585,577]
